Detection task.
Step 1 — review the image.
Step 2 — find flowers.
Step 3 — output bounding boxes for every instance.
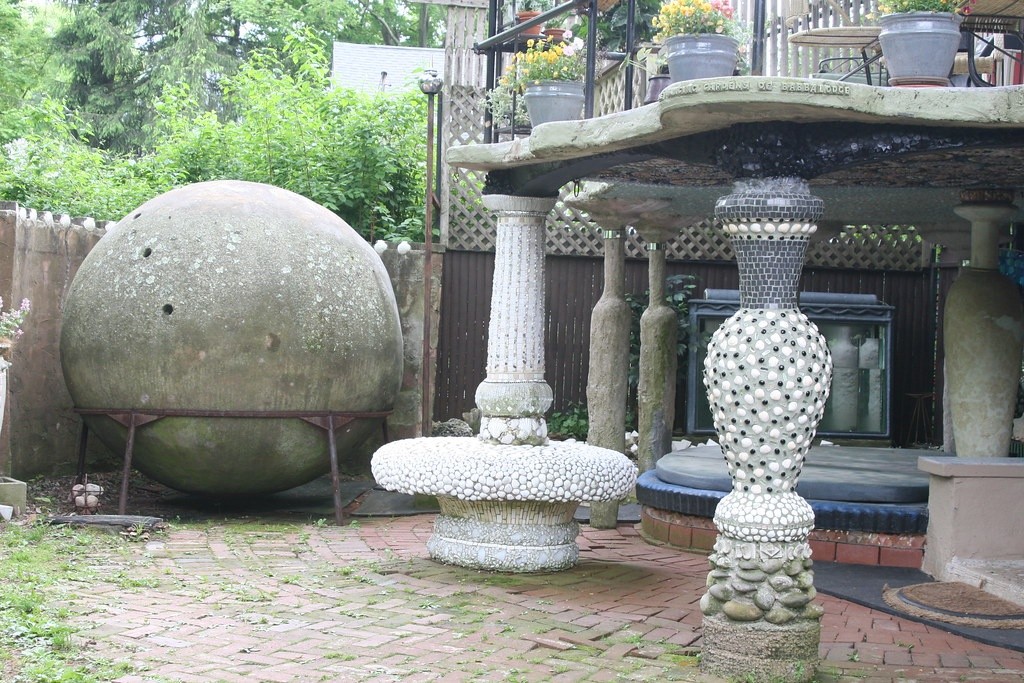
[652,1,745,45]
[859,0,976,26]
[497,35,586,91]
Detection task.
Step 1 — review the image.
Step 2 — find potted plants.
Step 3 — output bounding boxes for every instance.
[515,0,548,36]
[618,47,672,105]
[543,10,571,41]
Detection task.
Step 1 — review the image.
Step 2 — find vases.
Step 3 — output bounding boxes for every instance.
[876,12,964,86]
[524,80,585,129]
[663,34,739,84]
